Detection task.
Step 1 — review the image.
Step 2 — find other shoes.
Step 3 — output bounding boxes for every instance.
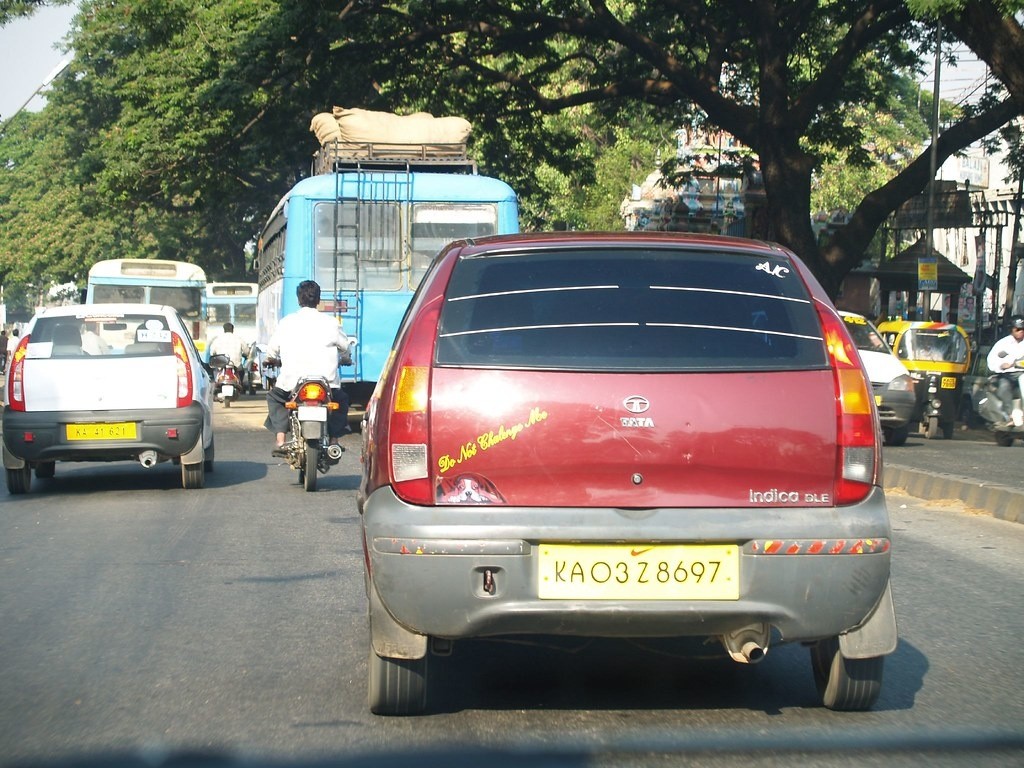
[331,441,346,452]
[271,443,287,455]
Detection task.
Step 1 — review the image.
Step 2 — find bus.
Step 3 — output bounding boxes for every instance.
[248,139,519,436]
[85,259,209,376]
[209,283,258,394]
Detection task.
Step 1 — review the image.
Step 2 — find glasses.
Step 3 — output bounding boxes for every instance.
[1016,327,1024,332]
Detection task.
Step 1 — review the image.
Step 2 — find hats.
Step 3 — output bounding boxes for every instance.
[1011,319,1024,329]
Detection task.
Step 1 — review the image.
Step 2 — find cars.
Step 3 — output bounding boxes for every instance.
[355,230,901,714]
[241,341,262,392]
[832,310,916,442]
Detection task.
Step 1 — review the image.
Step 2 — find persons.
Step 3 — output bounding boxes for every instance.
[80,322,109,356]
[943,284,976,323]
[0,329,20,375]
[986,314,1024,427]
[265,280,351,452]
[868,330,943,361]
[210,323,249,386]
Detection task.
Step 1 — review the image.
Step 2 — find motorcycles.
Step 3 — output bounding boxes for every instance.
[971,351,1024,447]
[262,356,354,493]
[212,360,244,409]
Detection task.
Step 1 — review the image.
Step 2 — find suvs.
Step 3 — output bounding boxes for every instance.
[1,303,213,495]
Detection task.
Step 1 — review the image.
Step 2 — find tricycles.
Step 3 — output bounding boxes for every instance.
[875,320,978,440]
